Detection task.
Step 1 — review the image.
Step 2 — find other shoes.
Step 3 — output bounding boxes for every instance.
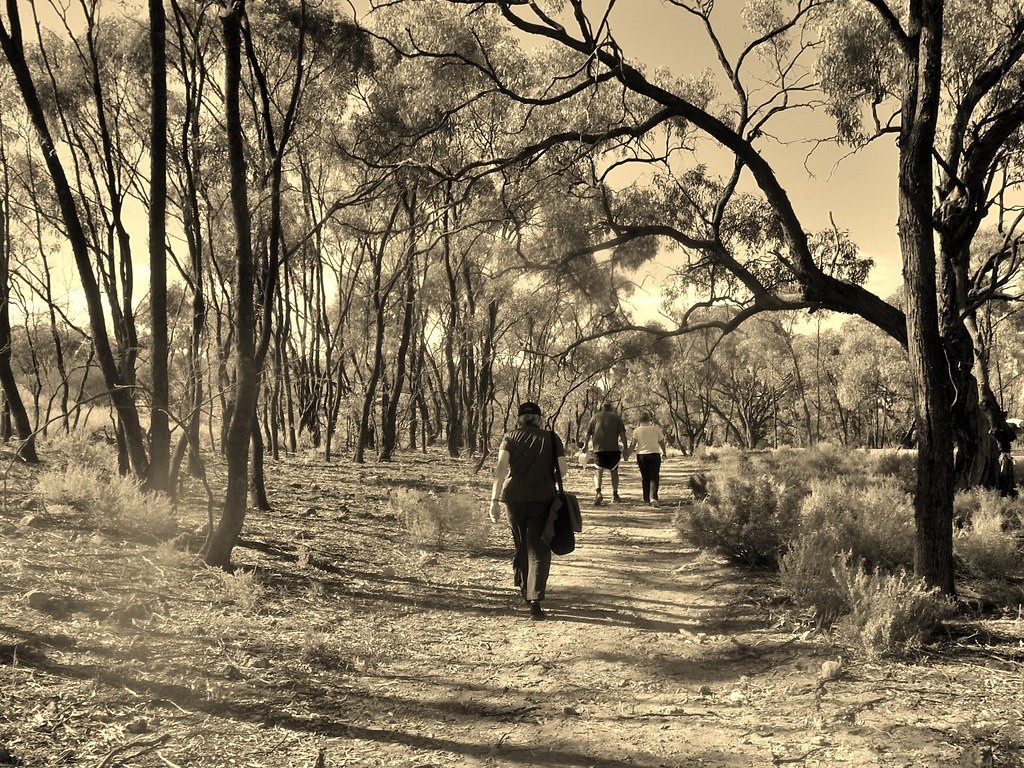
[521,588,531,603]
[530,601,547,620]
[650,498,659,508]
[594,493,603,505]
[612,495,620,503]
[643,501,650,506]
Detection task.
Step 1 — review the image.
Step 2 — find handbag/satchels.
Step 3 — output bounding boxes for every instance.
[550,490,575,555]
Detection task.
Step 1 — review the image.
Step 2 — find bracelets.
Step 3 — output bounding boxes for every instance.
[491,498,499,501]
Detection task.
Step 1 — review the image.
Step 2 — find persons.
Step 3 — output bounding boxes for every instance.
[625,414,667,507]
[489,402,568,611]
[583,403,628,504]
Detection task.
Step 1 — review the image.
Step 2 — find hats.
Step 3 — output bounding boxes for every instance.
[518,402,541,416]
